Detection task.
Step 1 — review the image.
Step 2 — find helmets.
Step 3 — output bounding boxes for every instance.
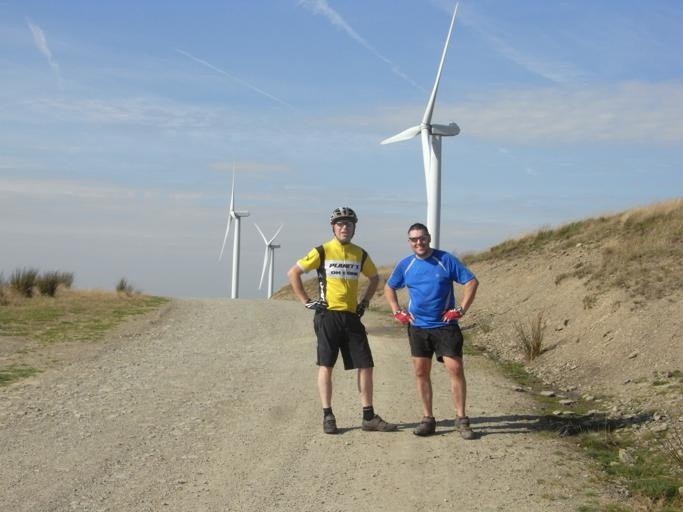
[330,207,358,222]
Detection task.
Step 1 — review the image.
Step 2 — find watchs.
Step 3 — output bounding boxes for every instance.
[456,306,465,315]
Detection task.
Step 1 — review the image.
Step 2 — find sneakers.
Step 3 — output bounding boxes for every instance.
[323,414,337,433]
[413,416,435,435]
[455,416,474,439]
[361,415,397,432]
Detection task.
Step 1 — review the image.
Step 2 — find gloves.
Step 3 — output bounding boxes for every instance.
[305,299,328,311]
[357,303,366,317]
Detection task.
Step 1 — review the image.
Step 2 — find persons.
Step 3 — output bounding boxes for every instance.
[384,223,479,440]
[287,207,396,433]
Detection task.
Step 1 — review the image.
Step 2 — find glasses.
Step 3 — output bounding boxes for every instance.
[335,221,354,226]
[409,237,429,242]
